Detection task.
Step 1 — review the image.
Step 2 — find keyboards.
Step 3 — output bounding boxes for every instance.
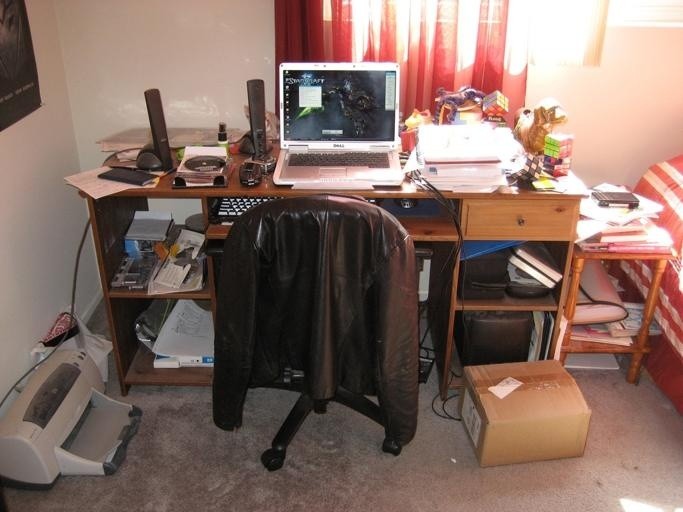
[214,197,286,222]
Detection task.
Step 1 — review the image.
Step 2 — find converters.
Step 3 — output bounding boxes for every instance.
[418,356,434,383]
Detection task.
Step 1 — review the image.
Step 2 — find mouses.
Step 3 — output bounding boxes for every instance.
[395,198,417,209]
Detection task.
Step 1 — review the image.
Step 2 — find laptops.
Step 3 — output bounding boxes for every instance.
[272,62,403,187]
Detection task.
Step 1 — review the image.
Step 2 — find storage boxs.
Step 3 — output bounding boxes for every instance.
[458,358,595,469]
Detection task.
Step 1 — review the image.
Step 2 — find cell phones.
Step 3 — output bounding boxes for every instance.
[239,163,262,186]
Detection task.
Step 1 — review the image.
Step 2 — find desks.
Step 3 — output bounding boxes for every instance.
[560,186,678,386]
[80,140,591,402]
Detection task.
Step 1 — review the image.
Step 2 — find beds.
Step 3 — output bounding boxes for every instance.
[609,154,683,417]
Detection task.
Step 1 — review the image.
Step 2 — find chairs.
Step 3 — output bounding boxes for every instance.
[212,194,419,471]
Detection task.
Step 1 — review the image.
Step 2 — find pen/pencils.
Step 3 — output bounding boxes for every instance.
[159,168,176,178]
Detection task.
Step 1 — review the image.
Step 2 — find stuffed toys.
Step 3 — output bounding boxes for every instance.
[432,86,487,126]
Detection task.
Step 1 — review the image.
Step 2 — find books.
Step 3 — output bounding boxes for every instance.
[510,238,564,283]
[569,323,633,347]
[147,258,207,295]
[563,352,620,370]
[605,301,663,338]
[526,311,556,363]
[509,253,556,293]
[411,122,510,194]
[573,181,676,252]
[97,168,157,186]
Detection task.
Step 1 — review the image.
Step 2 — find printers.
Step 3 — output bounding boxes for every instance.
[0,348,143,490]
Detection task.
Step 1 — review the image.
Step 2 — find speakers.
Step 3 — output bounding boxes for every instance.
[136,88,173,171]
[239,79,273,155]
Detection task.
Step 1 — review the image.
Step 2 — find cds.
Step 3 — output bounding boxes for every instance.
[184,155,225,171]
